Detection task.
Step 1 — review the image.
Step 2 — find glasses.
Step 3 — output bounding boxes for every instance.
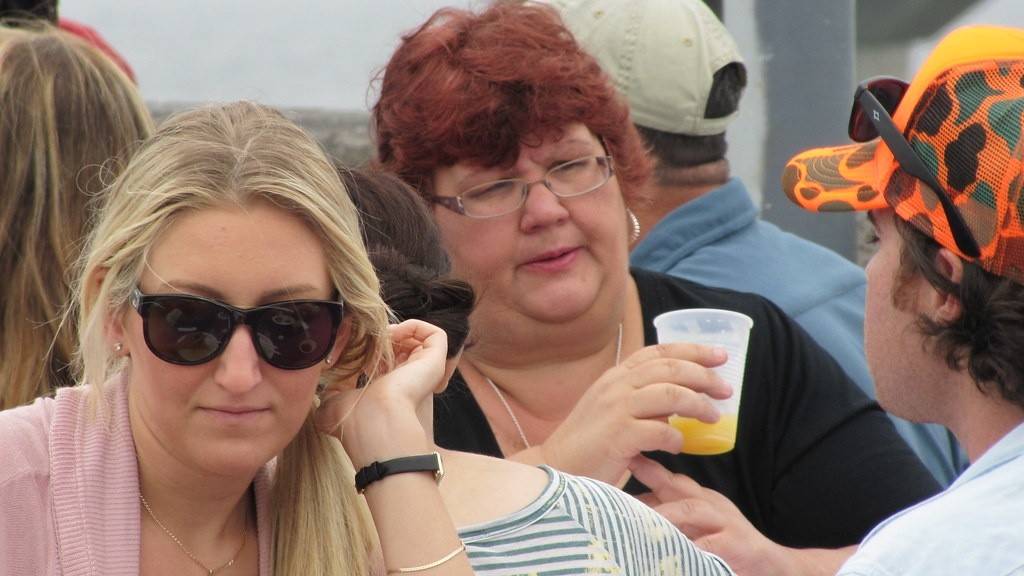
[848,75,984,258]
[121,271,347,371]
[413,132,616,220]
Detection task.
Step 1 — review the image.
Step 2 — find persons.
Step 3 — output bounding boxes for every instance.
[522,0,969,494]
[362,0,942,576]
[777,22,1024,576]
[0,0,136,87]
[334,164,740,576]
[0,99,479,576]
[1,16,159,411]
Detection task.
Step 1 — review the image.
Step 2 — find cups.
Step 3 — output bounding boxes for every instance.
[652,308,754,457]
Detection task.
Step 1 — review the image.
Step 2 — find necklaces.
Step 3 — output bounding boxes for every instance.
[484,321,623,451]
[137,490,250,575]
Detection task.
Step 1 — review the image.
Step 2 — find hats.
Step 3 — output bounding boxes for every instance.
[521,0,750,136]
[783,23,1024,288]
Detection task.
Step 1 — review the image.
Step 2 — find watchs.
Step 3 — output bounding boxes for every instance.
[354,450,445,496]
